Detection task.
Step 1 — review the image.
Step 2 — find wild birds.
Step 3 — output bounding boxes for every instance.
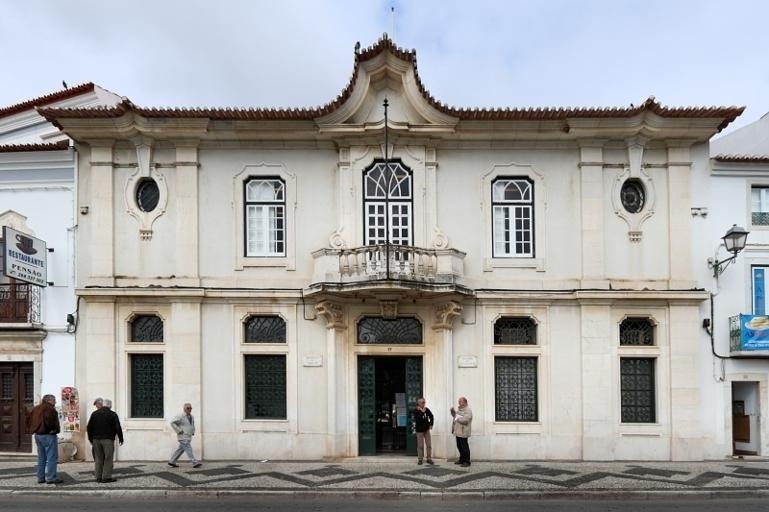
[62,79,69,91]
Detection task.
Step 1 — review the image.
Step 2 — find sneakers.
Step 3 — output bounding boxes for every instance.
[168,463,202,468]
[37,477,117,484]
[418,459,434,465]
[454,460,471,467]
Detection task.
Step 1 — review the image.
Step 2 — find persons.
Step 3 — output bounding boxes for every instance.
[414,398,435,464]
[166,403,203,468]
[90,397,114,467]
[450,397,472,467]
[85,399,123,483]
[25,394,62,483]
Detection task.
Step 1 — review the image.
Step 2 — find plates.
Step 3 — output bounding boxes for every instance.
[745,319,769,331]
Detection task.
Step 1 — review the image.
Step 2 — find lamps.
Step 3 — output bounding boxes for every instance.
[706,224,750,277]
